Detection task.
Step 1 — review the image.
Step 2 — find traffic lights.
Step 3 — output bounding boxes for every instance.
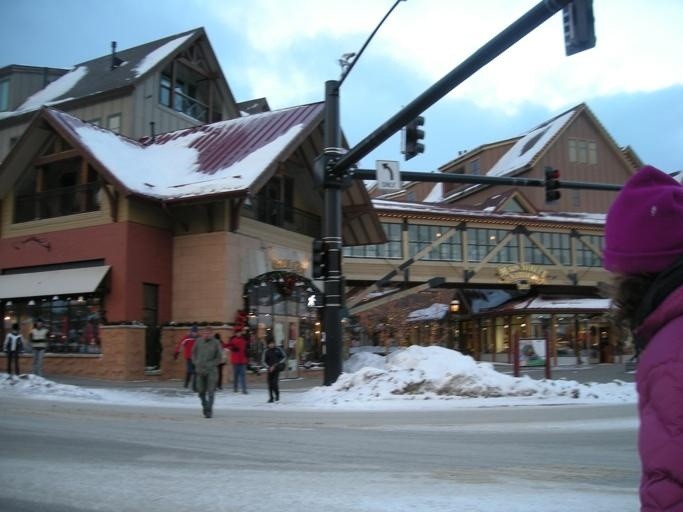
[546,166,561,203]
[405,116,425,157]
[314,241,329,277]
[305,292,325,309]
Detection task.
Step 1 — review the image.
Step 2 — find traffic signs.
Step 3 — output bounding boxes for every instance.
[376,159,401,189]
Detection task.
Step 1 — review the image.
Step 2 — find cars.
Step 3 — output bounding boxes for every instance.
[18,321,86,354]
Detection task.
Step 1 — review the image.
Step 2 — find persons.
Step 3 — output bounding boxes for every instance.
[25,318,50,377]
[602,165,683,512]
[173,327,288,418]
[3,323,26,376]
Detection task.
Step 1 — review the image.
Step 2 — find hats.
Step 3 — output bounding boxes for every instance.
[190,321,198,333]
[11,323,20,330]
